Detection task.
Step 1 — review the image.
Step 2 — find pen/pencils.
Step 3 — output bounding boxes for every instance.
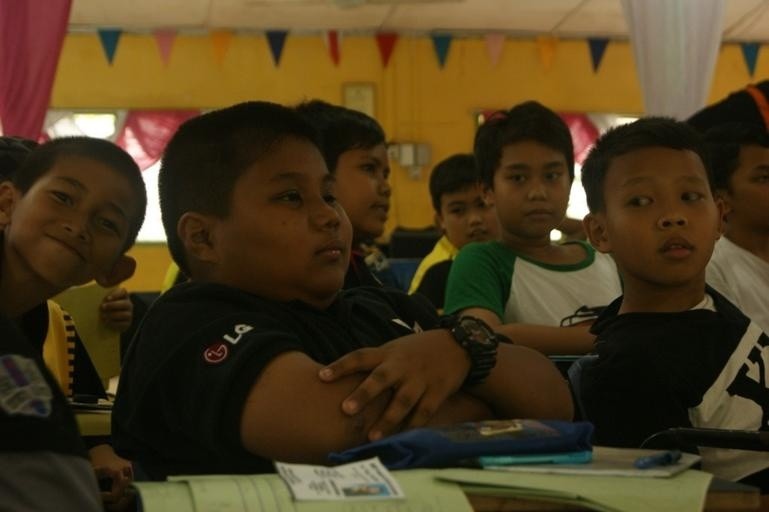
[633,448,681,470]
[477,450,593,465]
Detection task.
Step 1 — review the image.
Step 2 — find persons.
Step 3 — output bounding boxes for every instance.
[580,115,767,495]
[2,134,147,511]
[108,99,575,484]
[440,102,622,357]
[285,101,392,289]
[412,150,499,317]
[682,105,769,339]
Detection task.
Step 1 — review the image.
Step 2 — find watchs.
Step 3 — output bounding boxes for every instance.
[440,305,499,396]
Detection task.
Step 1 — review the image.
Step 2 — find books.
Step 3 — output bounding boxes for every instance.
[128,446,714,512]
